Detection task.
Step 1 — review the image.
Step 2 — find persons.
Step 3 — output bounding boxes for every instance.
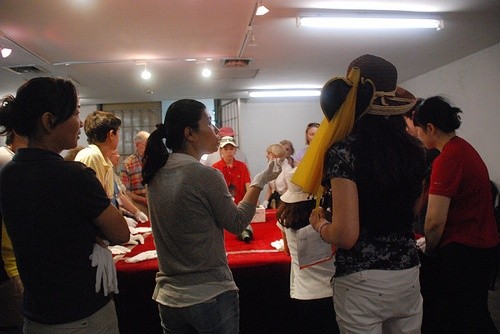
[277,166,337,334]
[140,98,283,334]
[212,135,251,206]
[309,54,431,334]
[257,139,300,209]
[0,95,29,169]
[1,76,131,334]
[74,110,122,201]
[414,95,500,334]
[119,130,151,214]
[65,146,148,227]
[297,123,320,162]
[206,126,247,167]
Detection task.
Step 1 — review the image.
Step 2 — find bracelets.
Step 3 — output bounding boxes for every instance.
[319,222,333,245]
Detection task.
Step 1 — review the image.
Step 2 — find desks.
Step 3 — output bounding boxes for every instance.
[112,208,425,334]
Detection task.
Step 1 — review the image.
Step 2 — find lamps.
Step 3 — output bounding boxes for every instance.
[247,26,257,47]
[295,9,443,31]
[254,1,270,17]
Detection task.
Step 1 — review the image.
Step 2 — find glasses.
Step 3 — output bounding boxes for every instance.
[308,123,320,128]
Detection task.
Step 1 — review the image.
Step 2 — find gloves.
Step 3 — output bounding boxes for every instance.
[129,227,152,235]
[120,233,144,245]
[249,158,282,190]
[416,236,427,253]
[89,240,119,296]
[125,249,157,263]
[134,212,148,224]
[108,245,131,255]
[262,200,269,209]
[123,216,138,228]
[271,239,284,251]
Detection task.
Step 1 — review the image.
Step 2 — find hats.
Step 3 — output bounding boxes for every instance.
[219,136,237,148]
[280,167,322,203]
[219,127,234,137]
[346,54,416,115]
